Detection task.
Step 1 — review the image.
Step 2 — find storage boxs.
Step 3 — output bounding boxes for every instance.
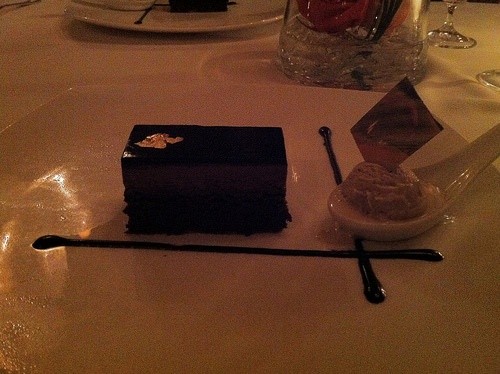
[119,124,292,236]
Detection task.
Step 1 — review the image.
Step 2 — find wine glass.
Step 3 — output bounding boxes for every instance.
[425,0,477,49]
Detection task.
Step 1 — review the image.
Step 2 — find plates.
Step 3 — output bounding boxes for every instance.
[64,0,298,33]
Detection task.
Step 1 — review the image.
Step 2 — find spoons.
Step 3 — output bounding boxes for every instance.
[327,123,500,242]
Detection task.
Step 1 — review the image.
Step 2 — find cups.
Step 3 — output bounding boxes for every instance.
[276,0,429,92]
